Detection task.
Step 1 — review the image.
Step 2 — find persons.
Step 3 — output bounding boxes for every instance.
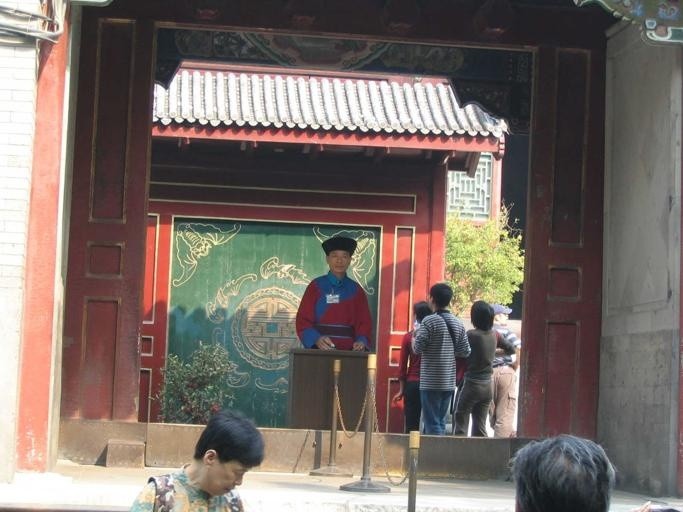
[412,283,472,435]
[505,431,616,512]
[127,408,265,511]
[393,301,433,431]
[454,300,516,437]
[488,303,521,438]
[296,234,372,352]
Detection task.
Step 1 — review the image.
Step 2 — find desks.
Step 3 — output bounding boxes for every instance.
[291,347,376,431]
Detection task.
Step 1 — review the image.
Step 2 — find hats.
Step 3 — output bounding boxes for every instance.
[322,236,358,253]
[491,304,512,315]
